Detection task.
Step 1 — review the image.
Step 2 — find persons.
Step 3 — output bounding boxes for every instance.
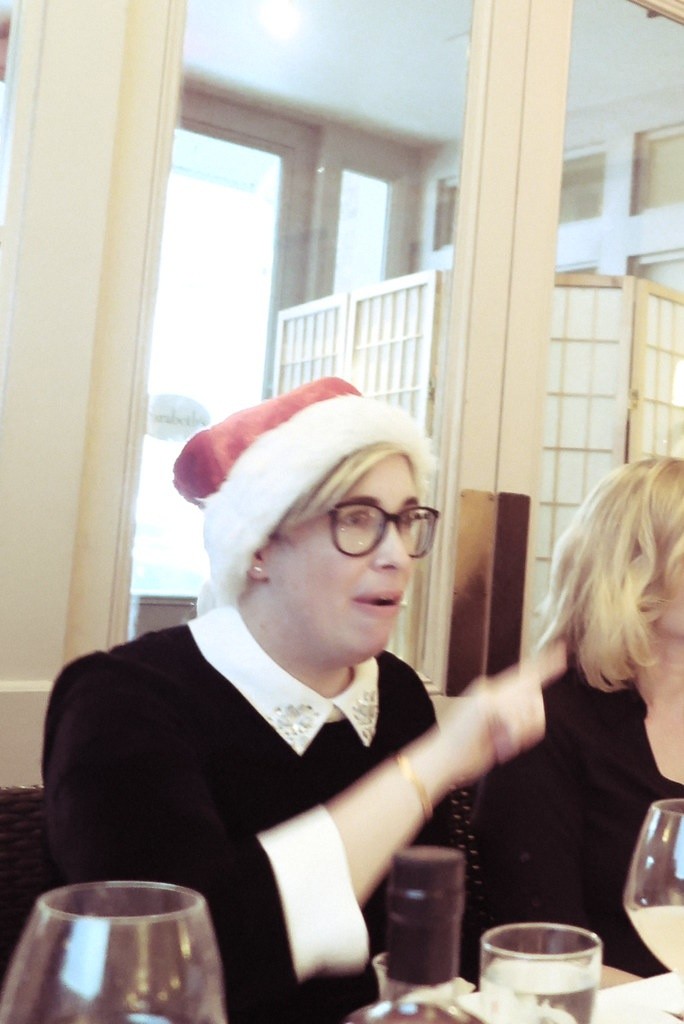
[35,374,567,1024]
[454,458,684,996]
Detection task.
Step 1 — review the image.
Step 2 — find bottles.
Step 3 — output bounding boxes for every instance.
[386,845,467,1001]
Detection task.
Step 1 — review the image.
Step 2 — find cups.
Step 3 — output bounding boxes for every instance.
[481,923,603,1024]
[624,798,684,972]
[0,881,225,1024]
[350,1000,485,1024]
[373,952,386,998]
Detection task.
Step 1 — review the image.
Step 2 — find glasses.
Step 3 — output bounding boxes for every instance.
[325,500,440,557]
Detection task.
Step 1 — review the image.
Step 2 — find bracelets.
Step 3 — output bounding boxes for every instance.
[392,746,436,826]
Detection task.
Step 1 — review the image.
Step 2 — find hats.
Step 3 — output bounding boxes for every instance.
[173,376,436,608]
